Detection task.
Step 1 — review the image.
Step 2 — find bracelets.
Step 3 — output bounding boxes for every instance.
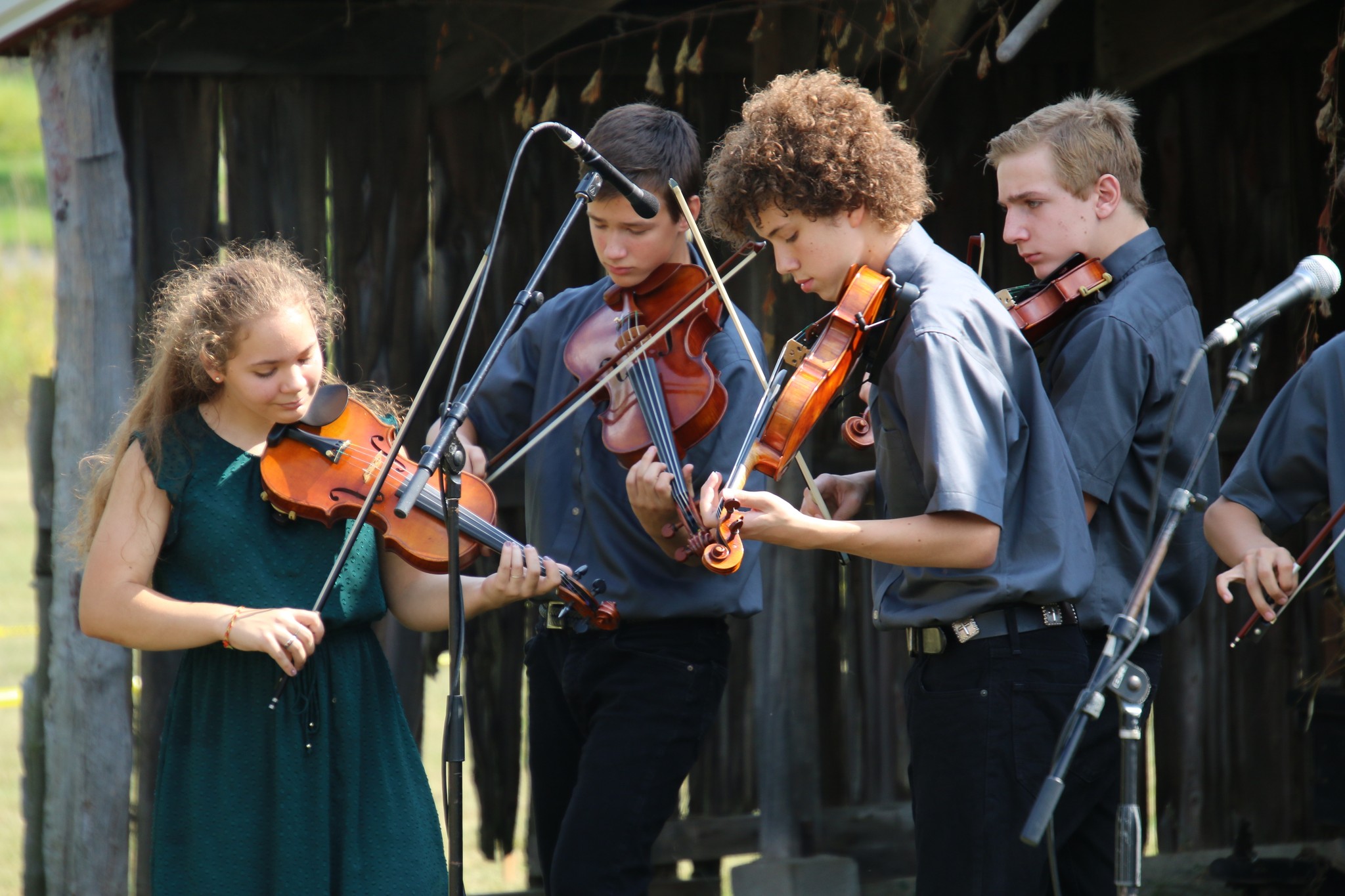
[222,606,245,650]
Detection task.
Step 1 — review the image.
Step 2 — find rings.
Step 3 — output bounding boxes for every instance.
[510,575,524,578]
[294,625,302,636]
[284,638,295,649]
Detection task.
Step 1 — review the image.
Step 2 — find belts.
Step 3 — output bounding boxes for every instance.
[906,603,1084,656]
[540,602,577,632]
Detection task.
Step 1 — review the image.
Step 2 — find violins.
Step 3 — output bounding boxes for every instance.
[840,250,1110,448]
[257,379,622,639]
[699,263,891,578]
[560,260,729,550]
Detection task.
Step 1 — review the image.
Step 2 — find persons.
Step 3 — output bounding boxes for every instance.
[424,104,773,896]
[694,68,1121,896]
[1203,332,1345,625]
[54,235,572,896]
[978,93,1217,896]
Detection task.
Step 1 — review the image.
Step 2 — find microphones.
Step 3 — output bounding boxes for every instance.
[1206,254,1342,352]
[554,124,659,218]
